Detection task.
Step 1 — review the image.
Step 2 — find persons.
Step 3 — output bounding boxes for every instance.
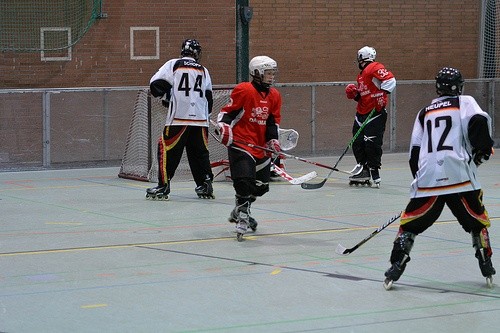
[145,39,215,201]
[383,67,497,291]
[345,45,396,188]
[216,56,285,242]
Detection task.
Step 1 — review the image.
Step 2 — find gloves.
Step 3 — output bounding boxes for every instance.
[345,84,358,99]
[215,122,233,146]
[472,146,491,167]
[375,89,388,112]
[159,92,171,108]
[266,139,286,159]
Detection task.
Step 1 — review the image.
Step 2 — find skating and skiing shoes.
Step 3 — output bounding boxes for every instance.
[229,195,258,232]
[349,161,372,186]
[195,174,215,199]
[234,198,251,241]
[475,248,496,287]
[370,167,381,189]
[145,180,170,200]
[383,254,410,289]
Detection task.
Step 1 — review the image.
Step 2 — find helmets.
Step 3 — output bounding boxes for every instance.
[181,39,202,64]
[358,46,376,62]
[249,55,277,88]
[435,67,464,95]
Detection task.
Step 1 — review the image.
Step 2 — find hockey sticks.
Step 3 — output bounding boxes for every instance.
[271,161,318,185]
[301,106,376,189]
[233,138,364,176]
[208,129,223,145]
[335,211,401,256]
